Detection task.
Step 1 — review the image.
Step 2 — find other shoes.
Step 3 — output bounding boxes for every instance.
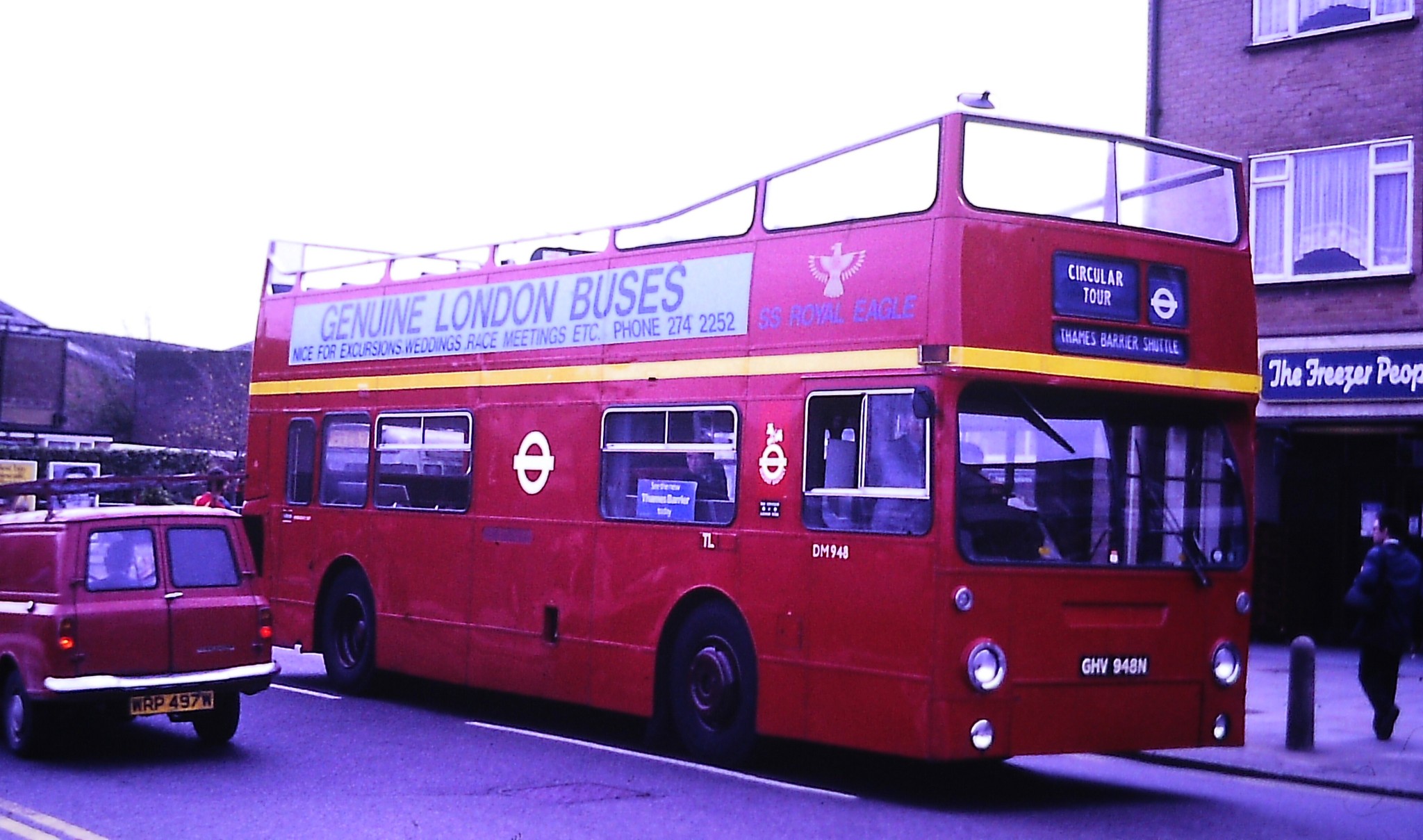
[1375,704,1398,739]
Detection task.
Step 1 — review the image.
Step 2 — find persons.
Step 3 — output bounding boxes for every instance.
[680,434,728,500]
[193,467,232,510]
[887,398,926,480]
[1344,509,1423,741]
[89,540,145,590]
[53,467,96,508]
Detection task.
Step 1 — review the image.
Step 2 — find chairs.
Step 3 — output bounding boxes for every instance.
[822,439,862,529]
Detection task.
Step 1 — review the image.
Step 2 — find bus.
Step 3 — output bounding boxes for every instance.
[239,86,1262,777]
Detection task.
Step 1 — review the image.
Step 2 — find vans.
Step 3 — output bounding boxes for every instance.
[1,474,282,758]
